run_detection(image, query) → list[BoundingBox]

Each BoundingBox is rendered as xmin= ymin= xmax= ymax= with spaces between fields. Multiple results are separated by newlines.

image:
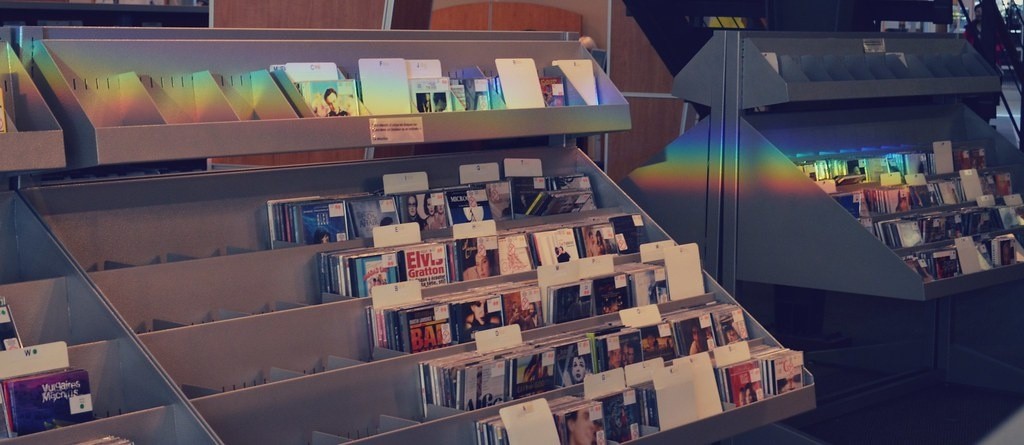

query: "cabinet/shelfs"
xmin=0 ymin=40 xmax=66 ymax=169
xmin=15 ymin=26 xmax=817 ymax=445
xmin=617 ymin=30 xmax=1023 ymax=427
xmin=0 ymin=189 xmax=222 ymax=445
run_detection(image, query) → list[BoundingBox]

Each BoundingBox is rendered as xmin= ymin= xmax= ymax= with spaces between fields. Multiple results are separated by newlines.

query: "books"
xmin=796 ymin=137 xmax=1024 ymax=283
xmin=885 ymin=52 xmax=908 ymax=68
xmin=0 ymin=297 xmax=135 ymax=445
xmin=761 ymin=53 xmax=778 ymax=73
xmin=862 ymin=38 xmax=886 ymax=54
xmin=267 ymin=60 xmax=803 ymax=445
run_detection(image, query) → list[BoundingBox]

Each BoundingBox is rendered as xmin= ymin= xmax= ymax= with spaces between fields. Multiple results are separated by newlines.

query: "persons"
xmin=323 ymin=87 xmax=352 ymax=117
xmin=965 ymin=3 xmax=1024 ymax=73
